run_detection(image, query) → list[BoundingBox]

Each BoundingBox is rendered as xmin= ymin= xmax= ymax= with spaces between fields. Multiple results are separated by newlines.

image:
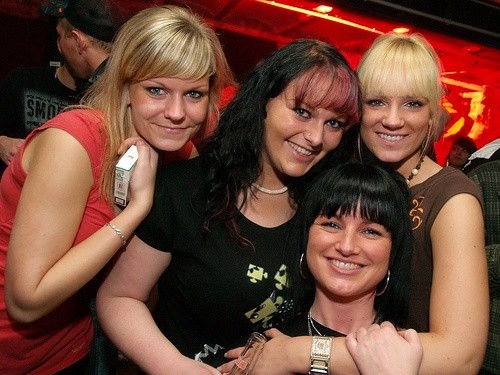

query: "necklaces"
xmin=308 ymin=311 xmax=312 ymax=336
xmin=310 ymin=309 xmax=321 ymax=336
xmin=405 ymin=152 xmax=425 ymax=185
xmin=251 ymin=183 xmax=288 ymax=195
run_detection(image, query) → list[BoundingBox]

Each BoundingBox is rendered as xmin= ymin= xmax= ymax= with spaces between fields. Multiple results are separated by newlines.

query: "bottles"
xmin=228 ymin=332 xmax=267 ymax=375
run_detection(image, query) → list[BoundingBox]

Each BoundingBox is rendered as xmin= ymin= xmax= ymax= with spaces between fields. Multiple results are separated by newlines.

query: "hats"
xmin=39 ymin=1 xmax=117 ymax=42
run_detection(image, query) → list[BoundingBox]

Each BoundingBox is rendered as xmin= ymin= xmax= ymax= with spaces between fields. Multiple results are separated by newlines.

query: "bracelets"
xmin=106 ymin=222 xmax=126 ymax=246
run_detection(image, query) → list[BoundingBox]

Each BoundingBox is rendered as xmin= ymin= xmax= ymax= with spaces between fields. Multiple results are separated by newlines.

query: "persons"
xmin=96 ymin=40 xmax=361 ymax=375
xmin=217 ymin=33 xmax=485 ymax=375
xmin=0 ymin=1 xmax=116 ymax=178
xmin=0 ymin=4 xmax=237 ymax=375
xmin=423 ymin=110 xmax=500 ymax=375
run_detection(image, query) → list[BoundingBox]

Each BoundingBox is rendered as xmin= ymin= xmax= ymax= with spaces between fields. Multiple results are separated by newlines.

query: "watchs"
xmin=309 ymin=336 xmax=334 ymax=375
